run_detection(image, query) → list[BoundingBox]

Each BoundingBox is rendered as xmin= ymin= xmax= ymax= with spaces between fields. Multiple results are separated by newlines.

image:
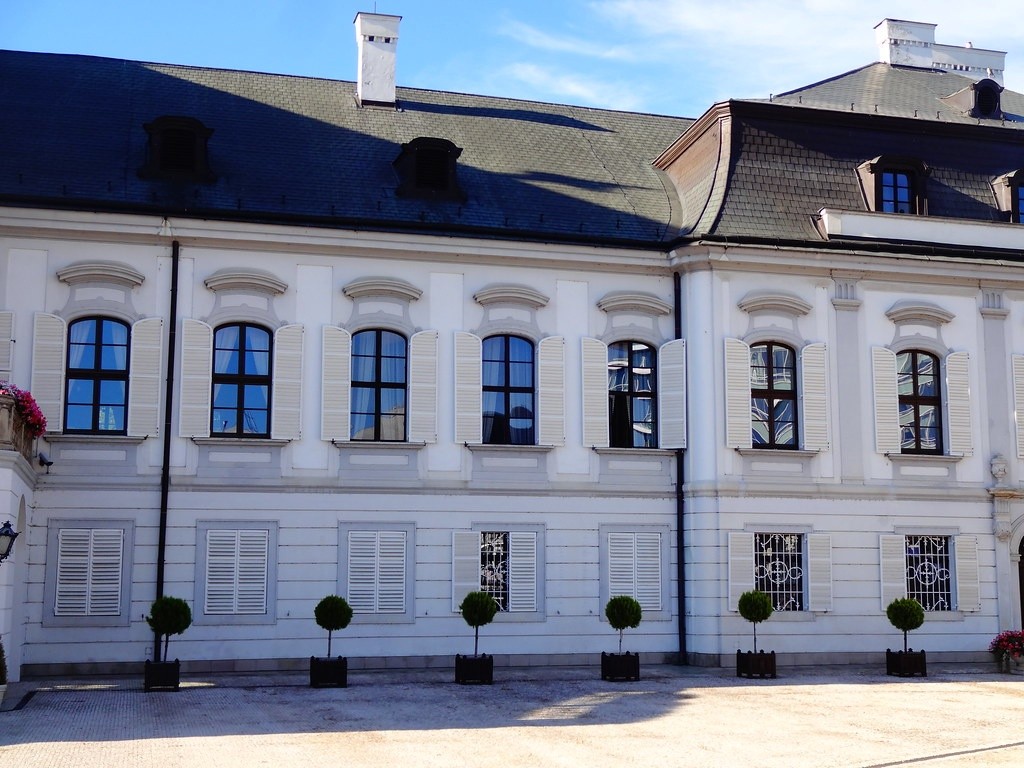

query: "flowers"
xmin=0 ymin=378 xmax=47 ymax=438
xmin=987 ymin=630 xmax=1024 ymax=664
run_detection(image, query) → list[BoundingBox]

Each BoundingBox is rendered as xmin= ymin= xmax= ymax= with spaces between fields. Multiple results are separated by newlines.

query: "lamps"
xmin=0 ymin=519 xmax=22 ymax=567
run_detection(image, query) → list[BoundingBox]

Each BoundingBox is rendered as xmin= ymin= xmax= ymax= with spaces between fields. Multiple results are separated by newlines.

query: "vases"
xmin=0 ymin=394 xmax=33 ymax=465
xmin=1002 ymin=653 xmax=1024 ymax=675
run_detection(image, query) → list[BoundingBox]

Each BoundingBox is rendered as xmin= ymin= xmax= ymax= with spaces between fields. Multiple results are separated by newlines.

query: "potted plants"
xmin=454 ymin=591 xmax=497 ymax=685
xmin=0 ymin=634 xmax=8 ymax=705
xmin=886 ymin=597 xmax=927 ymax=677
xmin=144 ymin=596 xmax=193 ymax=691
xmin=601 ymin=595 xmax=642 ymax=681
xmin=310 ymin=593 xmax=354 ymax=687
xmin=736 ymin=589 xmax=776 ymax=678
